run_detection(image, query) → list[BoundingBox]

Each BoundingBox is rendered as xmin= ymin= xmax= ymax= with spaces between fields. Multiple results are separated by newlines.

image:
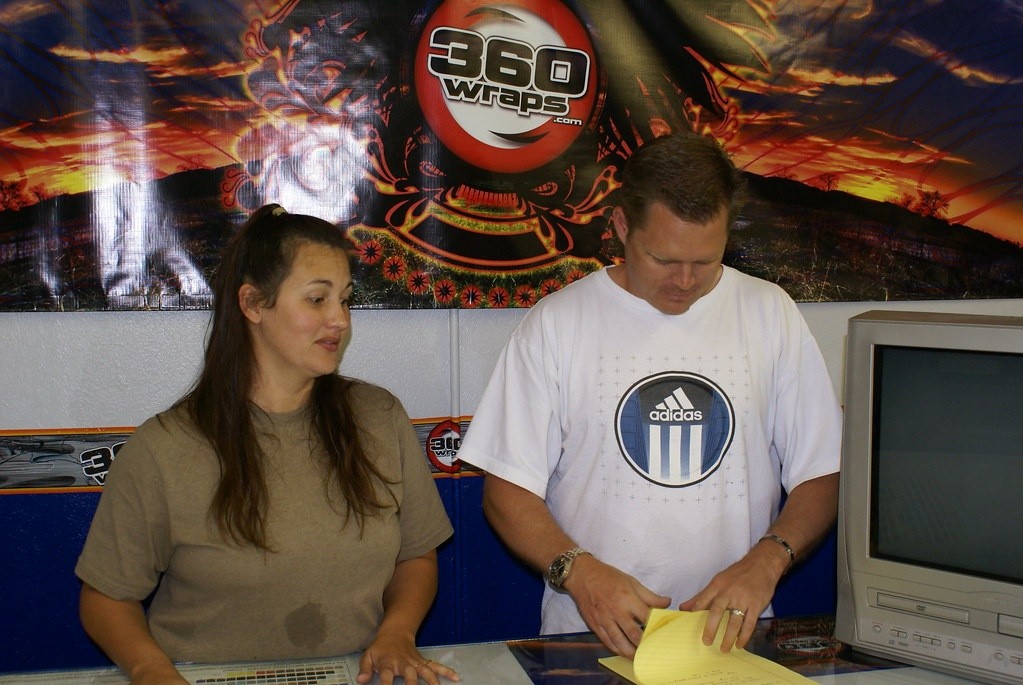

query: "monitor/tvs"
xmin=834 ymin=308 xmax=1023 ymax=685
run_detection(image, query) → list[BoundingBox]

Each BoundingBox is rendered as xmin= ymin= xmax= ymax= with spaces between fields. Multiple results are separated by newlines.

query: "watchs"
xmin=548 ymin=547 xmax=592 ymax=594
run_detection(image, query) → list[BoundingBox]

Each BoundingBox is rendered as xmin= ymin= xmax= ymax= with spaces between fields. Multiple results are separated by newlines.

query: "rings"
xmin=414 ymin=661 xmax=423 ymax=668
xmin=731 ymin=609 xmax=745 ymax=617
xmin=423 ymin=659 xmax=432 ymax=665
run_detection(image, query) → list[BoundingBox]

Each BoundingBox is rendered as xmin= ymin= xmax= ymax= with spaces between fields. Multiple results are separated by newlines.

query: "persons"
xmin=74 ymin=202 xmax=457 ymax=685
xmin=450 ymin=130 xmax=842 ymax=661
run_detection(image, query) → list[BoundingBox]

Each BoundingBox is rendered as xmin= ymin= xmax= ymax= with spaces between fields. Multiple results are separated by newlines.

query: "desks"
xmin=0 ymin=615 xmax=987 ymax=685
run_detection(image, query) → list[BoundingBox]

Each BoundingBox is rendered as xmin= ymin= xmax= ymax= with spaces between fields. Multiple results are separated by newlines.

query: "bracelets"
xmin=759 ymin=534 xmax=794 ymax=566
